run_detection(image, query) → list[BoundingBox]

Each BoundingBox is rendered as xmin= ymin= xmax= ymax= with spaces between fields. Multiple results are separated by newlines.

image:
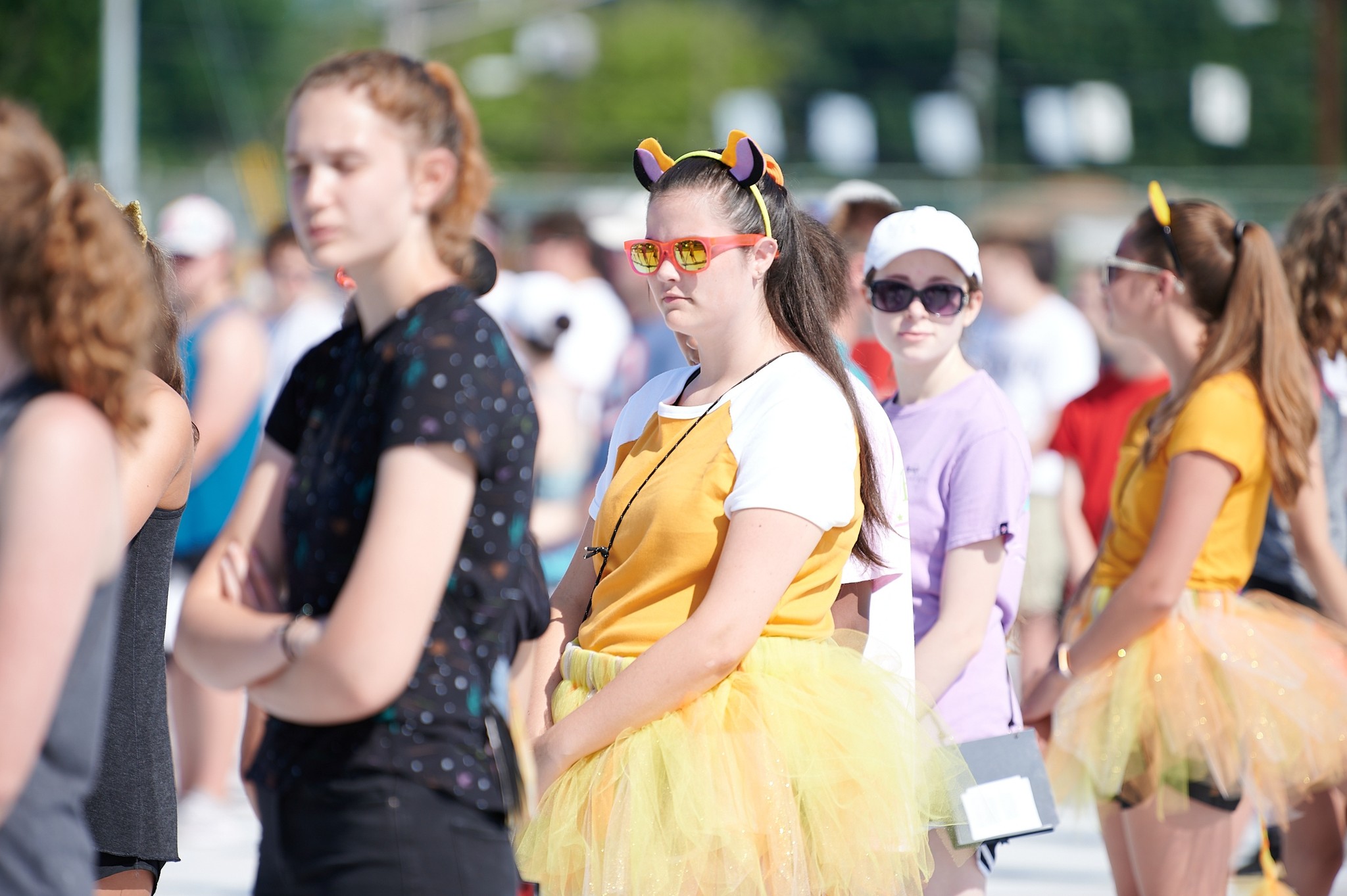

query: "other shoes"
xmin=161 ymin=793 xmax=261 ymax=890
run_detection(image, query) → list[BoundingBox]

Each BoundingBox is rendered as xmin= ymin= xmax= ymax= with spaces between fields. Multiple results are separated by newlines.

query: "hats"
xmin=152 ymin=196 xmax=233 ymax=257
xmin=864 ymin=205 xmax=983 ymax=285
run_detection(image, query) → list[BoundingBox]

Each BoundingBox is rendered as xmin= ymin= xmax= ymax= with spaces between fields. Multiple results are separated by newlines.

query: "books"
xmin=937 ymin=727 xmax=1061 ymax=848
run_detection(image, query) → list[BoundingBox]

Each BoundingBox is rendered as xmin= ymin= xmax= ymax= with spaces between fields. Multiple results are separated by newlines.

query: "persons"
xmin=1228 ymin=177 xmax=1347 ymax=896
xmin=856 ymin=208 xmax=1033 ymax=896
xmin=518 ymin=146 xmax=886 ymax=896
xmin=173 ymin=46 xmax=545 ymax=896
xmin=1012 ymin=197 xmax=1323 ymax=896
xmin=796 ymin=181 xmax=1173 ymax=710
xmin=439 ymin=183 xmax=699 ymax=722
xmin=0 ymin=99 xmax=352 ymax=896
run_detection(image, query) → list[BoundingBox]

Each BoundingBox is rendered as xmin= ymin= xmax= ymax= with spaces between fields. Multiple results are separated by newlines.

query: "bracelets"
xmin=278 ymin=614 xmax=313 ymax=669
xmin=1054 ymin=640 xmax=1079 ymax=683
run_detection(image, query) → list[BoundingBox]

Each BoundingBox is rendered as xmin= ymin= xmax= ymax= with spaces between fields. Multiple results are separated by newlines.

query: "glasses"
xmin=1102 ymin=255 xmax=1190 ymax=300
xmin=870 ymin=279 xmax=972 ymax=318
xmin=625 ymin=231 xmax=778 ymax=279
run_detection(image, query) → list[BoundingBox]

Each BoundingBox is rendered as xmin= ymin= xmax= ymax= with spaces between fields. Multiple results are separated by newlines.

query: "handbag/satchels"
xmin=938 ymin=729 xmax=1061 ymax=850
